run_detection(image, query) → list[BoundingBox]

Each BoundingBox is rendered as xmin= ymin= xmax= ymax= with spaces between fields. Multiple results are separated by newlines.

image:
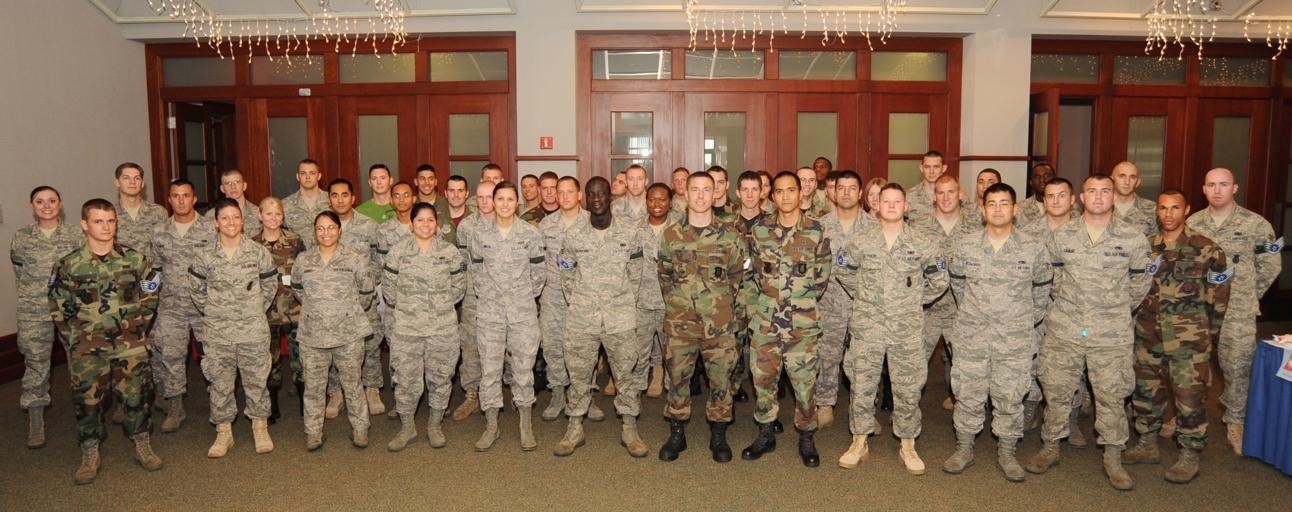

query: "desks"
xmin=1241 ymin=333 xmax=1292 ymax=478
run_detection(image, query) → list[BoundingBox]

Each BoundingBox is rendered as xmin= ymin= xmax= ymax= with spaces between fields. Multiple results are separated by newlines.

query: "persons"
xmin=517 ymin=176 xmax=538 ymax=213
xmin=826 ymin=172 xmax=838 ymax=208
xmin=141 ymin=180 xmax=216 ymax=433
xmin=456 ymin=184 xmax=496 ymax=419
xmin=251 ymin=196 xmax=306 ymax=423
xmin=1123 ymin=190 xmax=1230 ymax=482
xmin=375 ymin=183 xmax=416 ymax=276
xmin=325 ymin=179 xmax=387 ymax=420
xmin=612 ymin=166 xmax=647 ymax=237
xmin=1186 ymin=168 xmax=1282 ymax=454
xmin=705 ymin=166 xmax=736 ymax=217
xmin=819 ymin=169 xmax=877 ymax=426
xmin=756 ymin=171 xmax=776 ymax=214
xmin=522 ymin=171 xmax=556 ymax=227
xmin=1111 ymin=162 xmax=1159 ymax=237
xmin=554 ymin=177 xmax=648 ymax=458
xmin=189 ymin=198 xmax=279 ymax=458
xmin=609 ymin=170 xmax=628 ymax=197
xmin=414 ymin=164 xmax=454 ymax=240
xmin=863 ymin=176 xmax=884 ymax=216
xmin=11 ymin=189 xmax=86 ymax=449
xmin=905 ymin=151 xmax=969 ymax=228
xmin=1027 ymin=174 xmax=1153 ymax=490
xmin=668 ymin=166 xmax=688 ymax=214
xmin=381 ymin=203 xmax=466 ymax=452
xmin=469 ymin=181 xmax=546 ymax=452
xmin=729 ymin=169 xmax=772 ymax=234
xmin=944 ymin=182 xmax=1052 ymax=481
xmin=48 ymin=198 xmax=163 ymax=484
xmin=283 ymin=160 xmax=330 ymax=250
xmin=468 ymin=164 xmax=501 ymax=212
xmin=204 ymin=169 xmax=259 ymax=240
xmin=834 ymin=182 xmax=951 ymax=474
xmin=628 ymin=183 xmax=683 ymax=397
xmin=290 ymin=208 xmax=375 ymax=450
xmin=658 ymin=172 xmax=743 ymax=461
xmin=1021 ymin=177 xmax=1080 ymax=246
xmin=443 ymin=174 xmax=472 ymax=226
xmin=795 ymin=167 xmax=829 ymax=220
xmin=960 ymin=169 xmax=1027 ymax=229
xmin=111 ymin=163 xmax=168 ymax=254
xmin=356 ymin=163 xmax=397 ymax=222
xmin=745 ymin=170 xmax=832 ymax=467
xmin=812 ymin=157 xmax=832 ymax=190
xmin=540 ymin=175 xmax=590 ymax=421
xmin=906 ymin=176 xmax=983 ymax=365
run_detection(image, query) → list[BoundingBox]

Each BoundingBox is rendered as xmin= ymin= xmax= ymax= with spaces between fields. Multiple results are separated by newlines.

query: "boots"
xmin=325 ymin=390 xmax=345 ymax=419
xmin=388 ymin=405 xmax=398 ymax=419
xmin=1164 ymin=447 xmax=1201 ymax=484
xmin=474 ymin=407 xmax=500 ymax=452
xmin=996 ymin=444 xmax=1025 ymax=481
xmin=1120 ymin=432 xmax=1161 ymax=464
xmin=518 ymin=406 xmax=537 ymax=451
xmin=659 ymin=420 xmax=687 ymax=460
xmin=306 ymin=431 xmax=325 ymax=450
xmin=207 ymin=421 xmax=234 ymax=458
xmin=881 ymin=378 xmax=894 ymax=412
xmin=1226 ymin=423 xmax=1246 ymax=455
xmin=817 ymin=405 xmax=834 ymax=429
xmin=269 ymin=389 xmax=280 ymax=422
xmin=542 ymin=387 xmax=567 ymax=419
xmin=943 ymin=432 xmax=976 ymax=473
xmin=349 ymin=429 xmax=368 ymax=447
xmin=689 ymin=380 xmax=701 ymax=396
xmin=160 ymin=396 xmax=187 ymax=432
xmin=27 ymin=407 xmax=46 ymax=448
xmin=452 ymin=388 xmax=480 ymax=421
xmin=1023 ymin=401 xmax=1037 ymax=431
xmin=1159 ymin=415 xmax=1178 ymax=438
xmin=1026 ymin=438 xmax=1060 ymax=473
xmin=75 ymin=439 xmax=102 ymax=484
xmin=752 ymin=417 xmax=783 ymax=433
xmin=365 ymin=387 xmax=385 ymax=415
xmin=742 ymin=422 xmax=776 ymax=460
xmin=586 ymin=399 xmax=604 ymax=421
xmin=426 ymin=407 xmax=446 ymax=448
xmin=1068 ymin=407 xmax=1087 ymax=448
xmin=621 ymin=414 xmax=648 ymax=457
xmin=646 ymin=366 xmax=664 ymax=397
xmin=898 ymin=438 xmax=925 ymax=475
xmin=838 ymin=434 xmax=869 ymax=468
xmin=387 ymin=412 xmax=418 ymax=451
xmin=734 ymin=388 xmax=747 ymax=401
xmin=1103 ymin=444 xmax=1133 ymax=489
xmin=111 ymin=401 xmax=123 ymax=421
xmin=604 ymin=376 xmax=616 ymax=395
xmin=799 ymin=430 xmax=819 ymax=466
xmin=251 ymin=418 xmax=274 ymax=453
xmin=129 ymin=432 xmax=163 ymax=470
xmin=153 ymin=392 xmax=185 ymax=419
xmin=553 ymin=415 xmax=586 ymax=456
xmin=709 ymin=422 xmax=731 ymax=462
